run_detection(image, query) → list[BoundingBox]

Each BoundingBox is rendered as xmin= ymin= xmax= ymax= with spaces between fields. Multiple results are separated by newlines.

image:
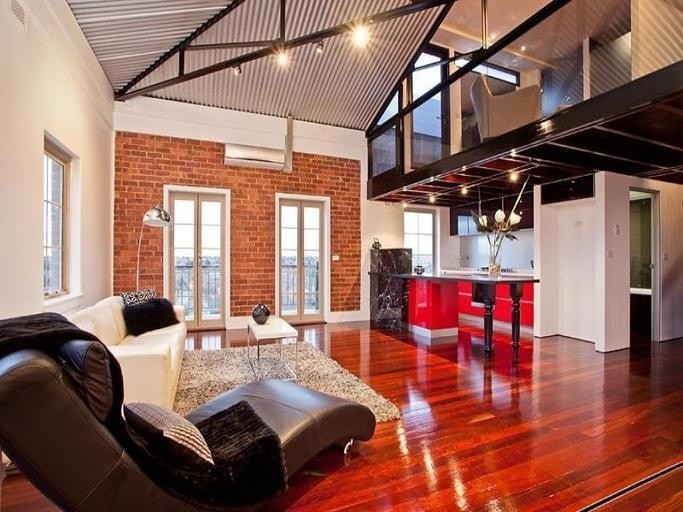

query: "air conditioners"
xmin=223 ymin=143 xmax=286 ymax=172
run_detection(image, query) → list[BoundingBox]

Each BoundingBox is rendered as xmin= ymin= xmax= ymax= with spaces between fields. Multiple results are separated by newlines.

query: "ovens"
xmin=471 ymin=281 xmax=496 ymax=309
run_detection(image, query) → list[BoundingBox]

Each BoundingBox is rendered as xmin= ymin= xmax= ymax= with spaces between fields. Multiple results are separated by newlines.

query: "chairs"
xmin=0 ymin=312 xmax=376 ymax=511
xmin=470 ymin=73 xmax=541 ymax=144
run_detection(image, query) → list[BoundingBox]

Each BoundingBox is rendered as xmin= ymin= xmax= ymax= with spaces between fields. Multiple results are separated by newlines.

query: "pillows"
xmin=124 ymin=402 xmax=216 ymax=477
xmin=121 ymin=287 xmax=159 ymax=308
xmin=122 ymin=298 xmax=180 ymax=337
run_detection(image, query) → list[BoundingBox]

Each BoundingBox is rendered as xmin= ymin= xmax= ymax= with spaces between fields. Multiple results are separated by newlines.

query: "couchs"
xmin=67 ymin=295 xmax=187 ymax=422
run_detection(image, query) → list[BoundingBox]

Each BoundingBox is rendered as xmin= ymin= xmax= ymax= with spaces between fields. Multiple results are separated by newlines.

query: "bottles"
xmin=414 ymin=266 xmax=425 ymax=275
xmin=372 ymin=242 xmax=381 ymax=250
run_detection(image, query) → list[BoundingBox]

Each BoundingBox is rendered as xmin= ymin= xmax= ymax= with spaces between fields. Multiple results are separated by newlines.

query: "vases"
xmin=487 ymin=237 xmax=502 ymax=278
xmin=251 ymin=303 xmax=270 ymax=324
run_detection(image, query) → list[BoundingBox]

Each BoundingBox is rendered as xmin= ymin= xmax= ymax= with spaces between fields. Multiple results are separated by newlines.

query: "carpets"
xmin=173 ymin=342 xmax=402 ymax=425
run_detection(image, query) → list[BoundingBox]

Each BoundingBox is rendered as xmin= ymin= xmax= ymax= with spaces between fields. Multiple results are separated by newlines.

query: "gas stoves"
xmin=474 ymin=267 xmax=513 ymax=272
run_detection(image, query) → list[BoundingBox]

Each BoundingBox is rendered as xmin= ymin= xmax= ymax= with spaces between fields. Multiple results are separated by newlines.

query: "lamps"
xmin=135 ymin=199 xmax=172 ymax=293
xmin=317 ymin=39 xmax=324 ymax=53
xmin=235 ymin=64 xmax=241 ymax=75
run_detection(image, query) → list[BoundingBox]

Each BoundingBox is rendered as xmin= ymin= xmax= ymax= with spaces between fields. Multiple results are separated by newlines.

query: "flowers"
xmin=470 ymin=176 xmax=530 ymax=267
